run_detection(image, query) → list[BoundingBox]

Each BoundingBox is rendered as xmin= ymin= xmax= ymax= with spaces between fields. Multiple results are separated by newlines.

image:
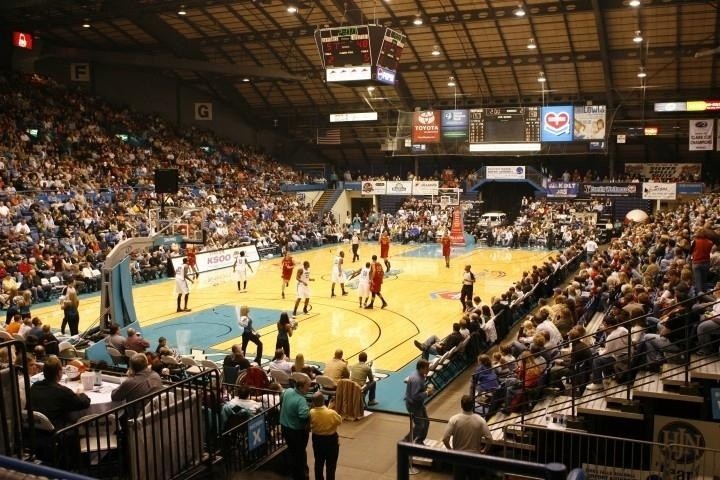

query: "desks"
xmin=30 ymin=370 xmax=126 ymax=407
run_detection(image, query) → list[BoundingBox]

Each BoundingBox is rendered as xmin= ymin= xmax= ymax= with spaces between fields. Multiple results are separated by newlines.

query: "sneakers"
xmin=696 ymin=350 xmax=713 ymax=356
xmin=360 ymin=305 xmax=362 ymax=308
xmin=388 ymin=262 xmax=390 ymax=267
xmin=177 ymin=309 xmax=184 ymax=312
xmin=642 ymin=363 xmax=656 ymax=371
xmin=342 ymin=292 xmax=348 ymax=295
xmin=184 ymin=308 xmax=191 ymax=311
xmin=387 ymin=266 xmax=389 ymax=272
xmin=543 ymin=387 xmax=560 ymax=393
xmin=352 ymin=260 xmax=355 ymax=262
xmin=303 ymin=309 xmax=309 ymax=314
xmin=381 ymin=303 xmax=387 ymax=309
xmin=357 ymin=255 xmax=359 ymax=259
xmin=286 ymin=283 xmax=288 ymax=287
xmin=447 ymin=263 xmax=449 ymax=268
xmin=243 ymin=289 xmax=247 ymax=292
xmin=331 ymin=294 xmax=336 ymax=298
xmin=238 ymin=290 xmax=243 ymax=293
xmin=255 ymin=358 xmax=262 ymax=366
xmin=446 ymin=263 xmax=447 ymax=268
xmin=365 ymin=304 xmax=373 ymax=309
xmin=282 ymin=293 xmax=285 ymax=299
xmin=292 ymin=313 xmax=296 ymax=318
xmin=364 ymin=302 xmax=367 ymax=306
xmin=368 ymin=400 xmax=378 ymax=406
xmin=586 ymin=382 xmax=605 ymax=390
xmin=463 ymin=304 xmax=467 ymax=312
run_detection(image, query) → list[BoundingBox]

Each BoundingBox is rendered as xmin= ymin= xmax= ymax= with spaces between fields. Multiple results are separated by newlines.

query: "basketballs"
xmin=437 ymin=239 xmax=440 ymax=242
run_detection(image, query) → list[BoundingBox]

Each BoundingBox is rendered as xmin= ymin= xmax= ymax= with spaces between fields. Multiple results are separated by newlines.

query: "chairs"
xmin=12 ymin=333 xmax=24 ymax=340
xmin=38 ymin=196 xmax=48 ymax=200
xmin=403 ymin=376 xmax=409 ymax=400
xmin=196 ymin=359 xmax=224 ymax=378
xmin=181 ymin=358 xmax=208 ymax=377
xmin=92 ymin=268 xmax=101 ymax=278
xmin=41 ymin=277 xmax=56 ymax=295
xmin=424 ymin=357 xmax=441 ymax=393
xmin=432 ymin=346 xmax=460 ymax=378
xmin=107 ymin=348 xmax=129 ymax=368
xmin=57 ymin=195 xmax=66 ymax=201
xmin=315 ymin=375 xmax=336 ymax=400
xmin=436 ymin=336 xmax=470 ymax=370
xmin=0 ymin=330 xmax=11 ymax=341
xmin=471 ymin=247 xmax=587 ymax=357
xmin=161 ymin=355 xmax=188 ymax=379
xmin=50 ymin=276 xmax=67 ymax=294
xmin=271 ymin=370 xmax=291 ymax=388
xmin=336 ymin=379 xmax=368 ymax=409
xmin=291 ymin=372 xmax=315 ymax=393
xmin=82 ymin=268 xmax=92 ymax=280
xmin=429 ymin=352 xmax=454 ymax=386
xmin=21 ymin=409 xmax=56 ymax=453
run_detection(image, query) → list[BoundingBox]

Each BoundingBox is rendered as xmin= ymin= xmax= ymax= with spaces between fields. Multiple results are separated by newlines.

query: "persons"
xmin=294 ymin=260 xmax=315 ymax=314
xmin=0 ymin=189 xmax=83 ymax=352
xmin=520 ymin=194 xmax=612 ymax=217
xmin=280 ymin=374 xmax=310 ymax=477
xmin=154 ymin=112 xmax=176 ymax=171
xmin=350 ymin=353 xmax=378 ymax=406
xmin=282 ymin=251 xmax=295 ymax=298
xmin=323 ymin=349 xmax=349 ymax=404
xmin=177 ymin=121 xmax=328 ymax=183
xmin=351 ymin=263 xmax=372 ymax=308
xmin=165 ymin=185 xmax=200 ymax=245
xmin=239 ymin=307 xmax=263 ymax=366
xmin=123 ymin=329 xmax=154 ymax=360
xmin=401 ymin=359 xmax=432 ymax=447
xmin=233 ymin=251 xmax=254 ymax=292
xmin=470 ymin=290 xmax=716 ymax=413
xmin=276 ymin=313 xmax=293 ymax=359
xmin=378 ymin=232 xmax=390 ymax=274
xmin=24 ymin=357 xmax=91 ymax=468
xmin=688 ymin=229 xmax=711 ymax=292
xmin=332 ymin=251 xmax=349 ymax=296
xmin=175 ymin=258 xmax=194 ymax=312
xmin=158 ymin=338 xmax=166 ymax=352
xmin=351 ymin=232 xmax=360 ymax=262
xmin=162 ymin=347 xmax=180 ymax=368
xmin=221 ymin=385 xmax=280 ymax=428
xmin=291 ymin=355 xmax=322 ymax=378
xmin=511 ymin=218 xmax=687 ymax=289
xmin=341 ymin=200 xmax=453 ymax=241
xmin=197 ymin=183 xmax=343 ymax=253
xmin=308 ymin=392 xmax=342 ymax=479
xmin=269 ymin=350 xmax=294 ymax=381
xmin=441 ymin=232 xmax=454 ymax=267
xmin=111 ymin=353 xmax=163 ymax=422
xmin=472 ymin=220 xmax=514 ymax=246
xmin=105 ymin=325 xmax=125 ymax=361
xmin=542 ymin=168 xmax=714 ymax=184
xmin=413 ymin=311 xmax=479 ymax=364
xmin=331 ymin=165 xmax=481 ymax=192
xmin=197 ymin=379 xmax=228 ymax=412
xmin=688 ymin=191 xmax=720 ymax=229
xmin=573 ymin=118 xmax=604 ymax=138
xmin=223 ymin=346 xmax=259 ymax=374
xmin=442 ymin=394 xmax=492 ymax=479
xmin=460 ymin=265 xmax=475 ymax=311
xmin=710 ymin=229 xmax=720 ymax=298
xmin=651 ymin=200 xmax=688 ymax=220
xmin=184 ymin=246 xmax=199 ymax=281
xmin=366 ymin=255 xmax=387 ymax=309
xmin=83 ymin=188 xmax=169 ymax=288
xmin=1 ymin=61 xmax=154 ymax=188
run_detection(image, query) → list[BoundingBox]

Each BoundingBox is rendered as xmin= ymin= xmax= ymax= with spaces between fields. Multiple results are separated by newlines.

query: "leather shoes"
xmin=414 ymin=340 xmax=424 ymax=351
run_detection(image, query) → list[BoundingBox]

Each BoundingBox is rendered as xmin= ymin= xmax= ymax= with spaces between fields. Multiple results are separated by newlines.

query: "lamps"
xmin=447 ymin=76 xmax=456 ymax=87
xmin=177 ymin=4 xmax=187 ymax=15
xmin=367 ymin=86 xmax=375 ymax=91
xmin=636 ymin=66 xmax=647 ymax=78
xmin=527 ymin=37 xmax=536 ymax=49
xmin=632 ymin=30 xmax=643 ymax=43
xmin=432 ymin=45 xmax=441 ymax=56
xmin=81 ymin=23 xmax=92 ymax=28
xmin=286 ymin=2 xmax=297 ymax=13
xmin=414 ymin=19 xmax=422 ymax=25
xmin=537 ymin=71 xmax=546 ymax=82
xmin=384 ymin=0 xmax=392 ymax=2
xmin=515 ymin=4 xmax=525 ymax=17
xmin=629 ymin=0 xmax=641 ymax=7
xmin=242 ymin=77 xmax=250 ymax=82
